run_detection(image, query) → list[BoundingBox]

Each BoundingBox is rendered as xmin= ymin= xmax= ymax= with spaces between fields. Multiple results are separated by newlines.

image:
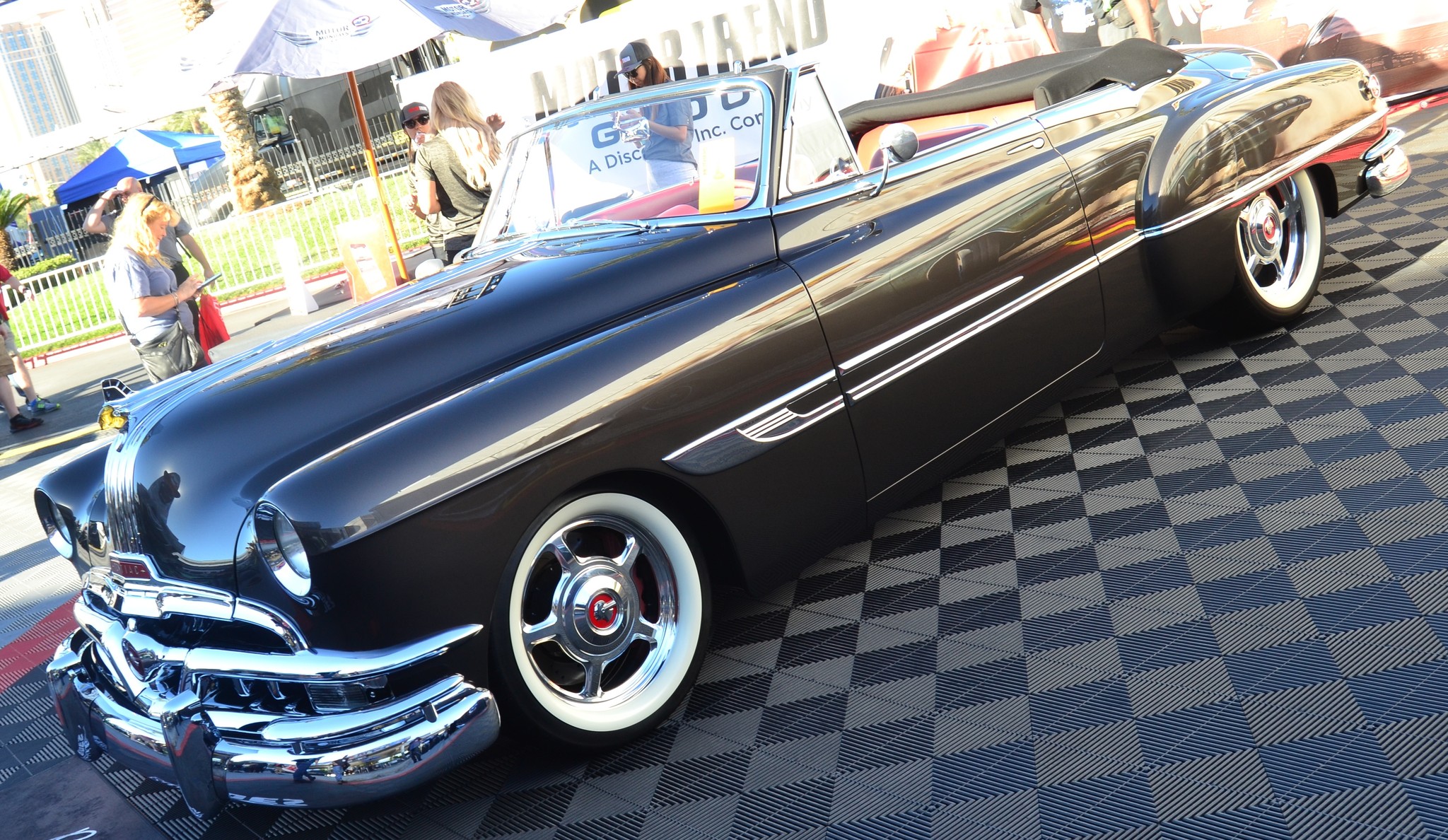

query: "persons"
xmin=81 ymin=177 xmax=216 ymax=368
xmin=147 ymin=470 xmax=182 ymax=523
xmin=398 ymin=101 xmax=506 ymax=267
xmin=102 ymin=193 xmax=213 ymax=387
xmin=0 ymin=256 xmax=60 ymax=415
xmin=412 ymin=82 xmax=504 ymax=268
xmin=609 ymin=41 xmax=700 ymax=189
xmin=0 ymin=319 xmax=44 ymax=433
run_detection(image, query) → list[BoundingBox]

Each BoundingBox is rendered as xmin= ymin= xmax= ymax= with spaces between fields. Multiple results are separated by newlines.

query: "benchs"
xmin=854 ymin=102 xmax=1036 ymax=180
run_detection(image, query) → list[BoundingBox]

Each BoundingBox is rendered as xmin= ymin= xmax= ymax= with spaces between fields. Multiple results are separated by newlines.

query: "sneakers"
xmin=26 ymin=397 xmax=50 ymax=411
xmin=30 ymin=400 xmax=61 ymax=416
xmin=9 ymin=415 xmax=44 ymax=433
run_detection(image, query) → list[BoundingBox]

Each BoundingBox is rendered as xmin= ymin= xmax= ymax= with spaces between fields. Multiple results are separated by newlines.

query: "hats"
xmin=399 ymin=102 xmax=430 ymax=129
xmin=613 ymin=43 xmax=653 ymax=78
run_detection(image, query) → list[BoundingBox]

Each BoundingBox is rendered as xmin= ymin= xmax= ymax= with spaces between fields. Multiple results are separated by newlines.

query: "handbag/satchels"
xmin=196 ymin=287 xmax=231 ymax=350
xmin=134 ymin=319 xmax=204 ymax=385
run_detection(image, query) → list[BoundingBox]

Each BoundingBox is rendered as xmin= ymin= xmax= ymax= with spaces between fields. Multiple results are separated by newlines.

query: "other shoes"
xmin=0 ymin=401 xmax=20 ymax=410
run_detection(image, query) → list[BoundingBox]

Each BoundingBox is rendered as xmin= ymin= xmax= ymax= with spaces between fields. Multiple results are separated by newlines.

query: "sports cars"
xmin=23 ymin=36 xmax=1405 ymax=812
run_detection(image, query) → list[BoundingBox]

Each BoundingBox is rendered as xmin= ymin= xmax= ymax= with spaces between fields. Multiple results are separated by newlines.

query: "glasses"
xmin=622 ymin=63 xmax=643 ymax=80
xmin=403 ymin=115 xmax=430 ymax=129
xmin=141 ymin=194 xmax=162 ymax=216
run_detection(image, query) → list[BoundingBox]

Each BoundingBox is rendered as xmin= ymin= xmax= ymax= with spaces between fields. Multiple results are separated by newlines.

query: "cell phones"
xmin=196 ymin=272 xmax=222 ymax=292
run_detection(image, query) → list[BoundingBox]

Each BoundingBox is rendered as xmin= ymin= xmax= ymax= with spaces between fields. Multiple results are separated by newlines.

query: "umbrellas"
xmin=52 ymin=128 xmax=227 ymax=207
xmin=201 ymin=0 xmax=594 ymax=282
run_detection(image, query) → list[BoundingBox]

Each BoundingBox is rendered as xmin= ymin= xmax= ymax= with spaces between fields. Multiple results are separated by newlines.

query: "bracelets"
xmin=172 ymin=290 xmax=180 ymax=307
